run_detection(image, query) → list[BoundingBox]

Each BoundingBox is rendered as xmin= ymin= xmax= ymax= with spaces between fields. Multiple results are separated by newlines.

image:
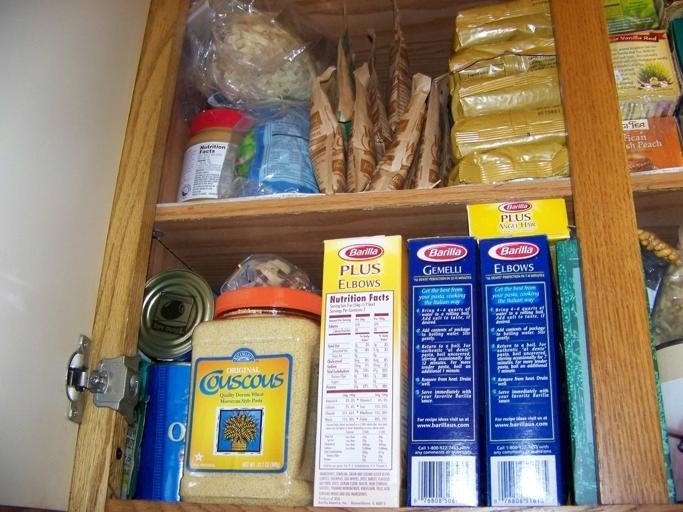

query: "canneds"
xmin=136 ymin=269 xmax=217 ymax=363
xmin=128 ymin=362 xmax=192 ymax=502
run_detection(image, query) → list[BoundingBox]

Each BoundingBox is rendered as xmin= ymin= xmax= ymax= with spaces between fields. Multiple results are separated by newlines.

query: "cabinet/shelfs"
xmin=59 ymin=0 xmax=682 ymax=511
